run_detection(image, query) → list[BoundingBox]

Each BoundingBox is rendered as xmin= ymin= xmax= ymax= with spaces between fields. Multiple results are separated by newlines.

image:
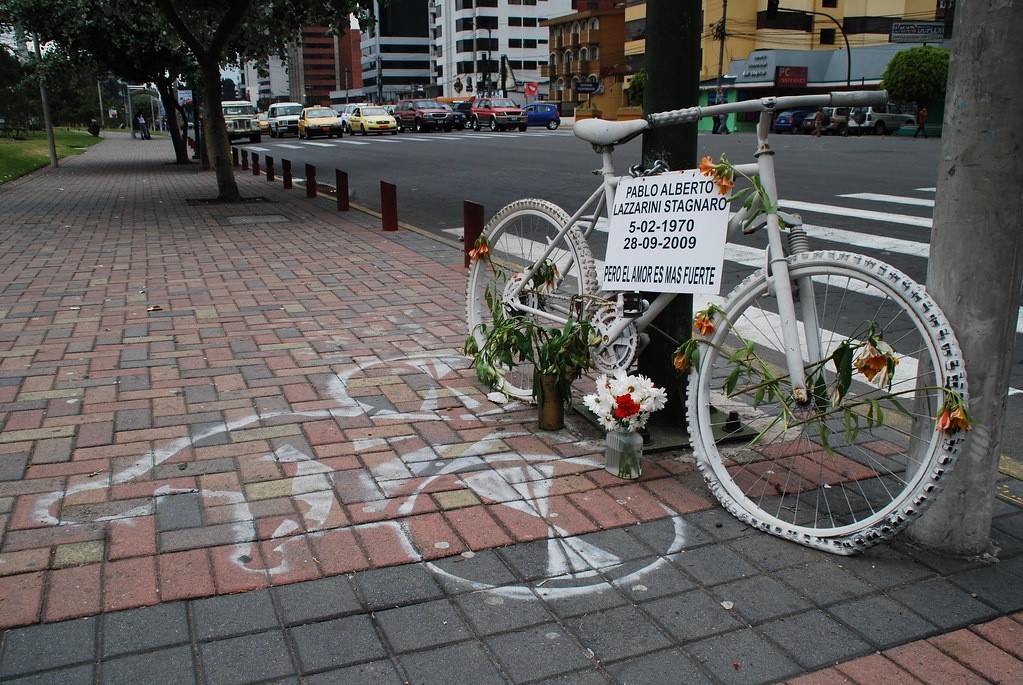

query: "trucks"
xmin=221 ymin=101 xmax=261 ymax=144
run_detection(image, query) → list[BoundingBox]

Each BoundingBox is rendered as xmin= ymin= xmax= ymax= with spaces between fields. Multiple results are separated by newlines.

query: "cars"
xmin=449 ymin=102 xmax=472 ymax=129
xmin=297 ymin=105 xmax=343 ymax=140
xmin=818 ymin=107 xmax=851 ymax=136
xmin=188 ymin=122 xmax=194 ymax=129
xmin=153 ymin=119 xmax=169 ymax=132
xmin=847 ymin=102 xmax=917 ymax=135
xmin=802 ymin=109 xmax=819 ymax=133
xmin=257 ymin=114 xmax=269 ymax=132
xmin=382 ymin=105 xmax=396 ymax=113
xmin=440 ymin=103 xmax=465 ymax=132
xmin=348 ymin=106 xmax=398 ymax=136
xmin=521 ymin=103 xmax=560 ymax=130
xmin=775 ymin=109 xmax=814 ymax=134
xmin=339 ymin=103 xmax=370 ymax=133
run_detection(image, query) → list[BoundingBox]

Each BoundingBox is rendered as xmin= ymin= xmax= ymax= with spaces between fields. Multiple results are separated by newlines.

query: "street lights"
xmin=709 ymin=21 xmax=726 ymax=86
xmin=469 ymin=21 xmax=491 ymax=80
xmin=363 ymin=54 xmax=382 ymax=102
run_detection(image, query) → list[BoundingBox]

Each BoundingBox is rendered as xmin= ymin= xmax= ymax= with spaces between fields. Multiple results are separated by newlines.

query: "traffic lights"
xmin=767 ymin=0 xmax=780 ymax=21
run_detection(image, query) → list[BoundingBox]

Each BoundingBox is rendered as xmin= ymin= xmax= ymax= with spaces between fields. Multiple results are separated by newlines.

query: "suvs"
xmin=469 ymin=97 xmax=527 ymax=132
xmin=391 ymin=99 xmax=452 ymax=133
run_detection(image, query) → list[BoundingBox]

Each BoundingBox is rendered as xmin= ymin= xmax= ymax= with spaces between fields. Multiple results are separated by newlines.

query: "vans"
xmin=268 ymin=102 xmax=304 ymax=138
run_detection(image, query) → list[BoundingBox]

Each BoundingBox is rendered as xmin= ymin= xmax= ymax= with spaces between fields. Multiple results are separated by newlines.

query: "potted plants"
xmin=464 ymin=313 xmax=601 ymax=430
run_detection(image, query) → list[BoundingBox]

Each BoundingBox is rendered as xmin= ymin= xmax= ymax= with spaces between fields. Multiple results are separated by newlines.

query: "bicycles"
xmin=465 ymin=91 xmax=969 ymax=555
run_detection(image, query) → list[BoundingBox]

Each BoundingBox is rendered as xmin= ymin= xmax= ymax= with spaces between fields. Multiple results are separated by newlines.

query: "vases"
xmin=606 ymin=426 xmax=643 ymax=479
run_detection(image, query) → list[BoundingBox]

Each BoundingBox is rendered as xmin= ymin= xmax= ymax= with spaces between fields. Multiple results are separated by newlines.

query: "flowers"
xmin=582 ymin=369 xmax=669 ymax=431
xmin=698 ymin=151 xmax=787 ymax=230
xmin=467 ymin=235 xmax=561 ymax=311
xmin=671 ymin=301 xmax=977 ymax=461
